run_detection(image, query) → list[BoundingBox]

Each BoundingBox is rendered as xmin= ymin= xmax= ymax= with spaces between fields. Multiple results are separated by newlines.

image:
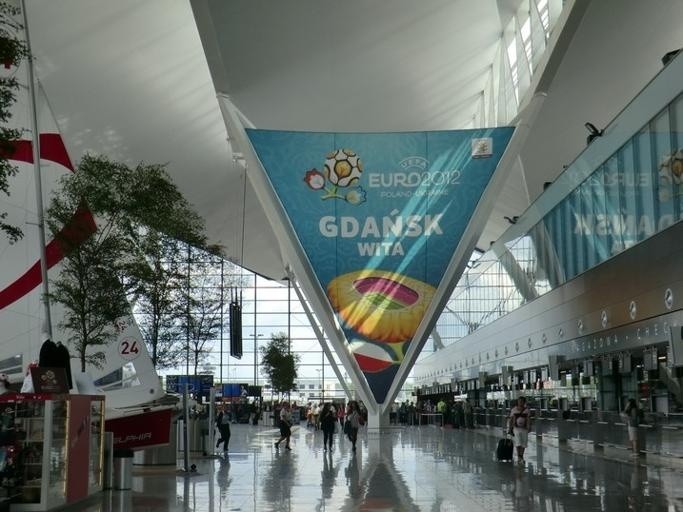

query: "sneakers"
xmin=285 ymin=446 xmax=291 ymax=450
xmin=629 ymin=453 xmax=640 ymax=458
xmin=517 ymin=459 xmax=525 ymax=465
xmin=630 ymin=419 xmax=638 ymax=428
xmin=275 ymin=443 xmax=278 ymax=449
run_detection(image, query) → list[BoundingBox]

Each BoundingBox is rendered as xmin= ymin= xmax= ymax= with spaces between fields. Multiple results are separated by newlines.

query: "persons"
xmin=211 ymin=396 xmax=474 ymax=453
xmin=319 ymin=450 xmax=337 ymax=498
xmin=624 ymin=398 xmax=640 ymax=457
xmin=508 ymin=397 xmax=531 ymax=465
xmin=625 ymin=458 xmax=643 ymax=512
xmin=509 ymin=469 xmax=534 ymax=512
xmin=215 ymin=453 xmax=232 ymax=492
xmin=344 ymin=453 xmax=362 ymax=499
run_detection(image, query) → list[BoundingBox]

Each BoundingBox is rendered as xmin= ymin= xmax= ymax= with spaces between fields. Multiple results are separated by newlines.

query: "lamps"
xmin=503 ymin=216 xmax=519 ymax=225
xmin=584 ymin=121 xmax=603 ymax=140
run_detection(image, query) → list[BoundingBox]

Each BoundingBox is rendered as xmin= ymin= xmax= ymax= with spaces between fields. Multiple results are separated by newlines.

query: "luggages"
xmin=495 ymin=426 xmax=513 ymax=461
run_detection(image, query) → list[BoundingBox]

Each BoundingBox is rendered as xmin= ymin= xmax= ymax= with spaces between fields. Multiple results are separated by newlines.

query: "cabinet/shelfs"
xmin=1 ymin=392 xmax=66 ymax=510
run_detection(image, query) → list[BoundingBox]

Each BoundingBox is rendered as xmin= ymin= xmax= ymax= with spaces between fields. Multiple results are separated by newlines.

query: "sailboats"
xmin=0 ymin=50 xmax=174 ymax=455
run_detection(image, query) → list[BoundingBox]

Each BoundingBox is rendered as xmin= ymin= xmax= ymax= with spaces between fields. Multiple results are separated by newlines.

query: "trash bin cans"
xmin=263 ymin=411 xmax=274 ymax=426
xmin=112 ymin=448 xmax=133 ymax=490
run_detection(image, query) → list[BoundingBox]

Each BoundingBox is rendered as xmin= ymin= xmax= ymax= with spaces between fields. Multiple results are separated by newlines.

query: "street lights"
xmin=315 ymin=368 xmax=323 ymax=399
xmin=249 ymin=332 xmax=263 ymax=398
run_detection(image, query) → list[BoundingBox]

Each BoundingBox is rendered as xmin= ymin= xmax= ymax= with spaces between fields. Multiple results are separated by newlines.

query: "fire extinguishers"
xmin=5 ymin=444 xmax=15 ymax=468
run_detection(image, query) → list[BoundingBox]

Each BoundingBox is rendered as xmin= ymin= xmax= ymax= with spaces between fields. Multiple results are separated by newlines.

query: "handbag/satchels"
xmin=344 ymin=415 xmax=352 ymax=434
xmin=507 ymin=426 xmax=515 ymax=436
